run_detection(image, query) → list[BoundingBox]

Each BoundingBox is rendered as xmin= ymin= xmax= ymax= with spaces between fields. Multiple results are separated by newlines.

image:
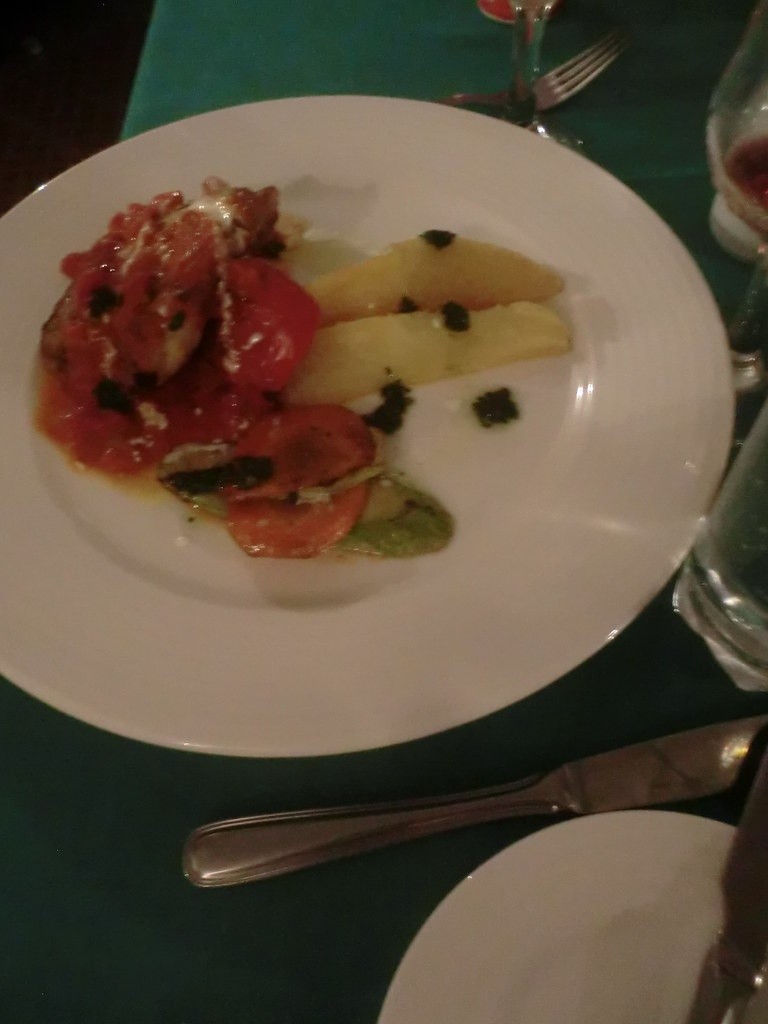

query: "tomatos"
xmin=227 ymin=401 xmax=378 ymax=563
xmin=203 ymin=258 xmax=321 ymax=399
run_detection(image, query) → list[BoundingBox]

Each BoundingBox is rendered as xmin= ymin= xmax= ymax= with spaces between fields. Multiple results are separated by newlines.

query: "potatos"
xmin=273 ymin=231 xmax=579 ymax=410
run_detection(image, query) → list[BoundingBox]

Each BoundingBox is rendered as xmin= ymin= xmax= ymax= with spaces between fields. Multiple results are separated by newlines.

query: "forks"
xmin=431 ymin=37 xmax=628 ymax=114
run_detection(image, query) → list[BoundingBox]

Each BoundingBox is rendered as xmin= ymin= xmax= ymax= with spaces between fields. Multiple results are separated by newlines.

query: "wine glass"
xmin=708 ymin=229 xmax=768 ymax=449
xmin=481 ymin=0 xmax=585 ymax=158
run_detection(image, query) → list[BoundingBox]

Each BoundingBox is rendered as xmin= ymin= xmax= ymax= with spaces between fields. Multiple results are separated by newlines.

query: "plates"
xmin=377 ymin=810 xmax=768 ymax=1024
xmin=0 ymin=91 xmax=733 ymax=761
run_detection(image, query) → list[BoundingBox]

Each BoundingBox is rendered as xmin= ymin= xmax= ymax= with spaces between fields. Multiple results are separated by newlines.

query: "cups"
xmin=699 ymin=3 xmax=768 ymax=267
xmin=671 ymin=390 xmax=768 ymax=701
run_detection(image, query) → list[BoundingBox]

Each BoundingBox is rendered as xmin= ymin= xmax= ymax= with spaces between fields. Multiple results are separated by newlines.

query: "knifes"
xmin=688 ymin=747 xmax=767 ymax=1022
xmin=179 ymin=715 xmax=767 ymax=909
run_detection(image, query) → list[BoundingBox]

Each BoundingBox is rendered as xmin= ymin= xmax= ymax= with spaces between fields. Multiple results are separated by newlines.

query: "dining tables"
xmin=1 ymin=0 xmax=768 ymax=1024
